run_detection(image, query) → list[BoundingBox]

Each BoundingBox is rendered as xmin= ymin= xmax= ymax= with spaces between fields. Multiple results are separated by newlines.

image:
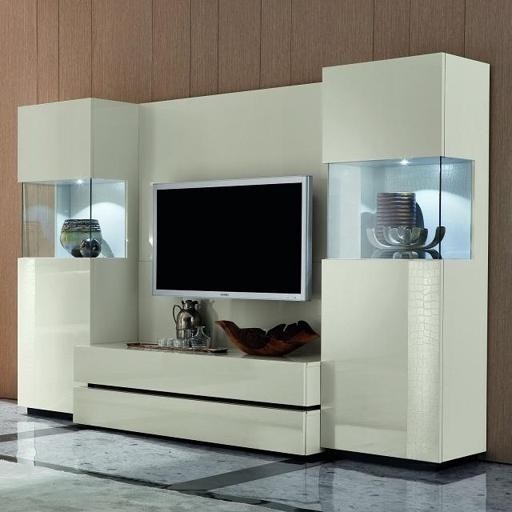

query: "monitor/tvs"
xmin=150 ymin=174 xmax=314 ymax=303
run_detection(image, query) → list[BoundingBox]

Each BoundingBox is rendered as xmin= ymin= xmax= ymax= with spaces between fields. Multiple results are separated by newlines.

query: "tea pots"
xmin=172 ymin=299 xmax=202 ymax=340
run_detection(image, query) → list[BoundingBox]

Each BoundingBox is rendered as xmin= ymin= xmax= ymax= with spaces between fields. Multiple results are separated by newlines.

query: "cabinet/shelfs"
xmin=17 ymin=51 xmax=490 ymax=463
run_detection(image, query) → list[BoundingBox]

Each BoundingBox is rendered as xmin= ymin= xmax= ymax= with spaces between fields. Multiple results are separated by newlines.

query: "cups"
xmin=173 ymin=340 xmax=183 ymax=349
xmin=167 ymin=338 xmax=173 ymax=348
xmin=183 ymin=340 xmax=189 ymax=349
